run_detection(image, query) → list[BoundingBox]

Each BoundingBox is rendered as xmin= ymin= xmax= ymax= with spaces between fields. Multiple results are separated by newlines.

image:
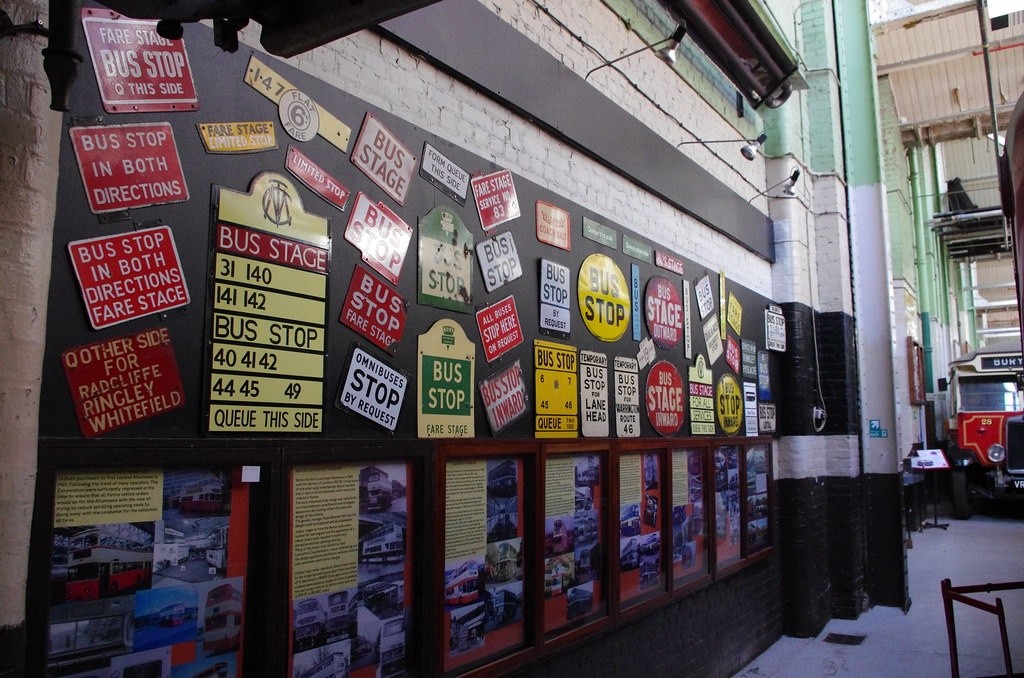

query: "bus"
xmin=64 ymin=445 xmax=768 ymax=676
xmin=937 ymin=342 xmax=1024 ymax=521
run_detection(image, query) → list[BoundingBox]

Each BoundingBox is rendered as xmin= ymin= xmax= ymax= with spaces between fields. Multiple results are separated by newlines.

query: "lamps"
xmin=747 ymin=169 xmax=801 ymax=204
xmin=584 ymin=20 xmax=687 ymax=82
xmin=677 ymin=131 xmax=769 ymax=163
